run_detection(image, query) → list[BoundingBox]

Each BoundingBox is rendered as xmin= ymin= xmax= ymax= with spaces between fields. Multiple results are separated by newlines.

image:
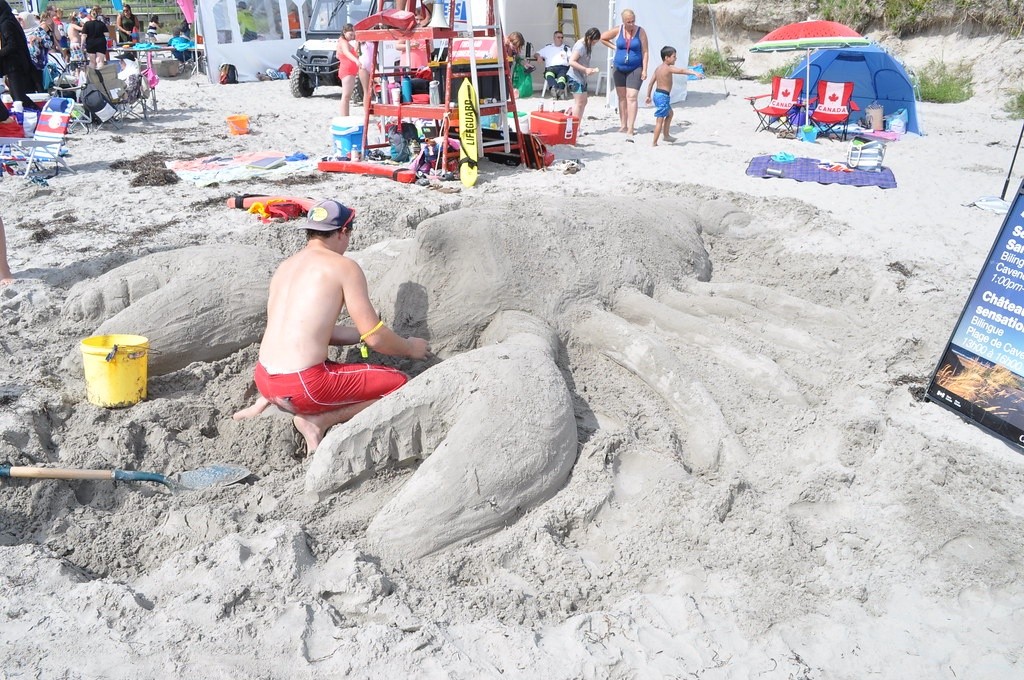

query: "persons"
xmin=237 ymin=1 xmax=258 ymax=42
xmin=0 ymin=217 xmax=16 ymax=285
xmin=600 ymin=8 xmax=649 ymax=136
xmin=533 ymin=31 xmax=572 ymax=100
xmin=396 ymin=8 xmax=435 ymax=70
xmin=354 ymin=40 xmax=380 ymax=113
xmin=0 ymin=0 xmax=141 ymax=139
xmin=168 ymin=17 xmax=200 ymax=63
xmin=646 ymin=46 xmax=703 ymax=146
xmin=504 ymin=32 xmax=534 ymax=71
xmin=231 ymin=201 xmax=434 ymax=458
xmin=147 ymin=15 xmax=161 ymax=43
xmin=334 ymin=23 xmax=363 ymax=116
xmin=564 ymin=27 xmax=601 ymax=132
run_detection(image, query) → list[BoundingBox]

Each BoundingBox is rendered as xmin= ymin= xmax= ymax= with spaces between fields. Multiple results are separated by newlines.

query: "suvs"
xmin=290 ymin=0 xmax=398 ymax=105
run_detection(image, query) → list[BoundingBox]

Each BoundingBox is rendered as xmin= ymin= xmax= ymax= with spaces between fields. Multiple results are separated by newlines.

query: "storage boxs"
xmin=329 ymin=116 xmax=364 ymax=157
xmin=0 ymin=92 xmax=50 ymax=111
xmin=431 ymin=62 xmax=510 ymax=104
xmin=530 ymin=111 xmax=580 ymax=146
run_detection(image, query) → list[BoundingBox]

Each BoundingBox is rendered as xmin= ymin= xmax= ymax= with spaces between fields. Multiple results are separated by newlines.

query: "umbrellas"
xmin=748 ymin=17 xmax=870 ymax=126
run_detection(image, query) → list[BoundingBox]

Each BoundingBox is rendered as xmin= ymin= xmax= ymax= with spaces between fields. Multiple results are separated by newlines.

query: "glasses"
xmin=556 ymin=36 xmax=563 ymax=39
xmin=341 ymin=207 xmax=355 ymax=231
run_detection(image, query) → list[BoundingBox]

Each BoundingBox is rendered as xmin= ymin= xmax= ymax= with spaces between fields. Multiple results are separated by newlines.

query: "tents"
xmin=776 ymin=46 xmax=921 ymax=136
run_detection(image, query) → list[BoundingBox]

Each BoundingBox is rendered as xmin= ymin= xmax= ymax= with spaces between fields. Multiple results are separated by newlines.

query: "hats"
xmin=297 ymin=199 xmax=353 ymax=231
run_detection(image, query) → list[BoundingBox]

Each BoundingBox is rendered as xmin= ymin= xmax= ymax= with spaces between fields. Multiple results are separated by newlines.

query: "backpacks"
xmin=218 ymin=63 xmax=240 ymax=85
xmin=526 ymin=130 xmax=554 ymax=167
xmin=387 ymin=123 xmax=419 ymax=163
xmin=80 ymin=84 xmax=118 ymax=124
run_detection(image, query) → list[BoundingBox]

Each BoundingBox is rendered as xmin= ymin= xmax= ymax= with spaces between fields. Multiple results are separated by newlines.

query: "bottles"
xmin=350 ymin=144 xmax=359 ymax=162
xmin=409 ymin=136 xmax=437 ymax=168
xmin=526 ymin=42 xmax=530 ymax=57
xmin=375 ymin=72 xmax=440 ymax=107
xmin=222 ymin=121 xmax=227 ymax=133
xmin=66 ymin=36 xmax=112 ymax=61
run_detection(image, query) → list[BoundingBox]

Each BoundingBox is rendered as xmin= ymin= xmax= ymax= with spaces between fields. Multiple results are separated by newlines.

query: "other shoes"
xmin=564 ymin=158 xmax=586 ymax=175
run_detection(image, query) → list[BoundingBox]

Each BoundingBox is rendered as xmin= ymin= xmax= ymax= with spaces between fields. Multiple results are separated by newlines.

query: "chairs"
xmin=809 ymin=80 xmax=861 ymax=142
xmin=744 ymin=76 xmax=804 ymax=138
xmin=595 ymin=57 xmax=613 ymax=96
xmin=53 ymin=51 xmax=160 ymax=135
xmin=541 ymin=78 xmax=570 ymax=100
xmin=0 ymin=97 xmax=76 ymax=179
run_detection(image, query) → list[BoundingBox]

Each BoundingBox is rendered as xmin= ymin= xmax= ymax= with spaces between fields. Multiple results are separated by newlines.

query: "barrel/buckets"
xmin=80 ymin=333 xmax=149 ymax=409
xmin=225 ymin=114 xmax=250 ymax=135
xmin=329 ymin=117 xmax=369 ymax=158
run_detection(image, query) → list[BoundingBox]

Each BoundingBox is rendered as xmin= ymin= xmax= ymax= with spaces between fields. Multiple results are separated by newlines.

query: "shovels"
xmin=0 ymin=461 xmax=252 ymax=498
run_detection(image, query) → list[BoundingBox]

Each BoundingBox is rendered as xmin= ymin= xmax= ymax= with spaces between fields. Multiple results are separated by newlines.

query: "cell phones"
xmin=524 ymin=66 xmax=535 ymax=73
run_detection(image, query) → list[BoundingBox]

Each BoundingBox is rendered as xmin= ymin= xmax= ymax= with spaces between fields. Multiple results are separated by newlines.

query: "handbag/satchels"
xmin=845 ymin=135 xmax=887 ymax=172
xmin=263 ymin=200 xmax=303 ymax=223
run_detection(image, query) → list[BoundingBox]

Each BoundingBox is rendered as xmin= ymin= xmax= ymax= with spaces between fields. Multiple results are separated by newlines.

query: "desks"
xmin=106 ymin=46 xmax=205 ymax=75
xmin=523 ymin=55 xmax=545 ymax=64
